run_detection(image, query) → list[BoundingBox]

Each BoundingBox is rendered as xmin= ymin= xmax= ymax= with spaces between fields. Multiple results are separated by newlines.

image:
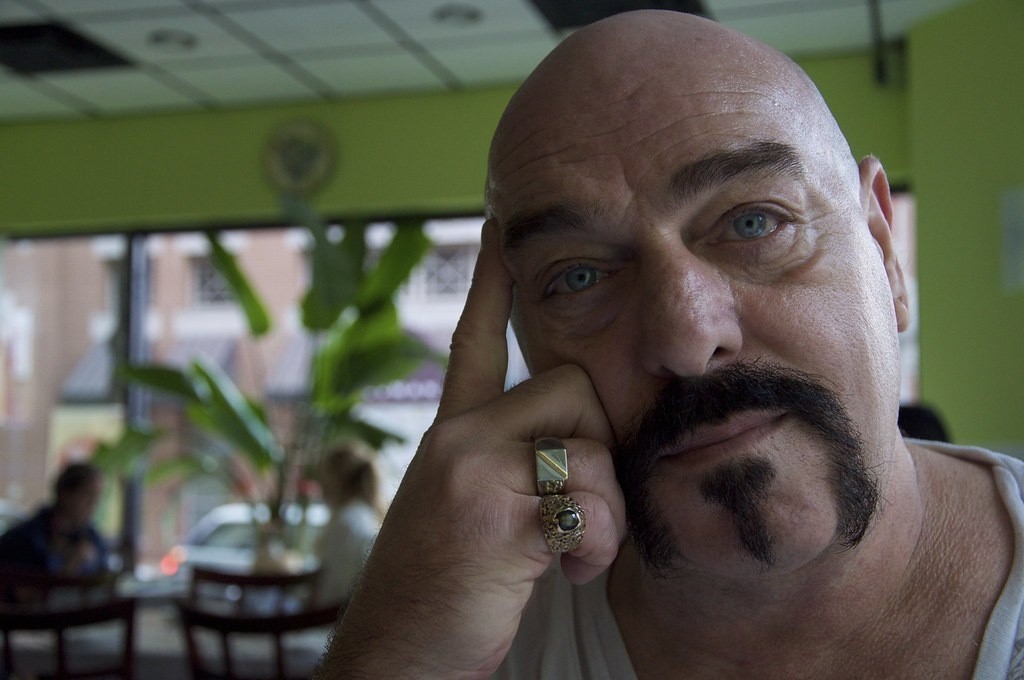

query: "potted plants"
xmin=87 ymin=196 xmax=453 ymax=578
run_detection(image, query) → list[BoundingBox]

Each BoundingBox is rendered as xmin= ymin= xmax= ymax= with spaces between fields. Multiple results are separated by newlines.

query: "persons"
xmin=325 ymin=9 xmax=1024 ymax=680
xmin=0 ymin=465 xmax=110 ymax=625
xmin=316 ymin=444 xmax=383 ymax=608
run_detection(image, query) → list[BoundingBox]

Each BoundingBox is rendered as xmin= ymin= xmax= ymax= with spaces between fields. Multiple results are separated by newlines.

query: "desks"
xmin=182 ymin=583 xmax=348 ymax=680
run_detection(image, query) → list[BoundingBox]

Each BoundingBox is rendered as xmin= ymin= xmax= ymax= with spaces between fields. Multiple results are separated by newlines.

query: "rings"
xmin=539 ymin=495 xmax=586 ymax=554
xmin=535 ymin=438 xmax=567 ymax=495
